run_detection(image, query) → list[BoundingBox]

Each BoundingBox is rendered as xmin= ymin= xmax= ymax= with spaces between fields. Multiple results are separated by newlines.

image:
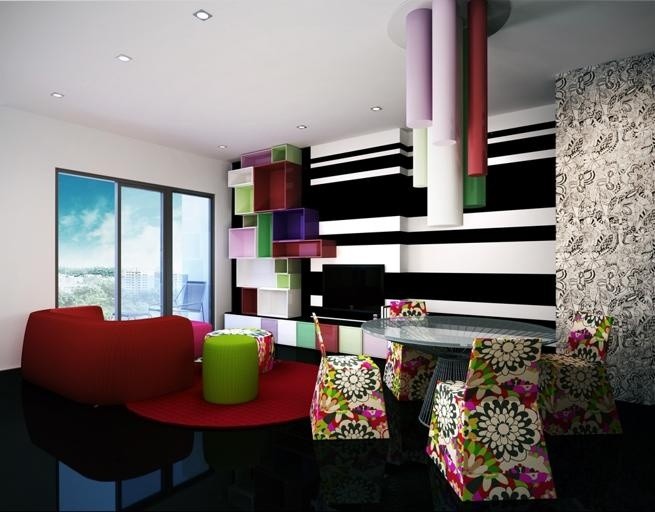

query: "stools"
xmin=202 ymin=333 xmax=259 ymax=405
xmin=189 ymin=319 xmax=213 ymax=361
xmin=207 ymin=329 xmax=275 ymax=375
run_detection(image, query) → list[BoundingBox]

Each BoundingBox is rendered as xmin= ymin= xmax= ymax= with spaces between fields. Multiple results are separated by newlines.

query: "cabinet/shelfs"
xmin=223 ymin=311 xmax=389 ymax=371
xmin=228 ymin=144 xmax=336 ymax=320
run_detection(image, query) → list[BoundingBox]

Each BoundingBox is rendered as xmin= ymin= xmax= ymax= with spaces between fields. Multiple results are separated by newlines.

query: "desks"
xmin=361 ymin=316 xmax=562 ymax=429
xmin=113 ymin=310 xmax=151 ymax=319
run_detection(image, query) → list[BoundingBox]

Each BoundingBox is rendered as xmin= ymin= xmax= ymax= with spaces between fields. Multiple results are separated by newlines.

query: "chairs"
xmin=309 ymin=312 xmax=391 ymax=441
xmin=149 ymin=281 xmax=206 ymax=322
xmin=536 ymin=310 xmax=623 ymax=435
xmin=426 ymin=338 xmax=557 ymax=502
xmin=382 ymin=300 xmax=437 ymax=401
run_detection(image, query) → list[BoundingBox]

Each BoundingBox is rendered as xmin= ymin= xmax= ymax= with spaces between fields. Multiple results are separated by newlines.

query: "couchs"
xmin=20 ymin=304 xmax=195 ymax=402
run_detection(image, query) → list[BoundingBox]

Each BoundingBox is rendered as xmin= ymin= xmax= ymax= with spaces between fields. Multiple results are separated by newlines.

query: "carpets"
xmin=121 ymin=357 xmax=319 ymax=429
